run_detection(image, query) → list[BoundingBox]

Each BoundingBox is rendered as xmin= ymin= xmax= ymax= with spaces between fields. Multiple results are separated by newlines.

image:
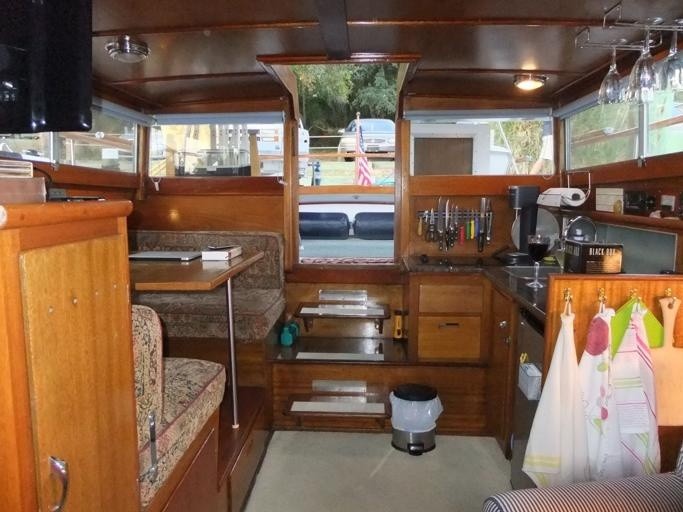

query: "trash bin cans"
xmin=389 ymin=383 xmax=435 ymax=455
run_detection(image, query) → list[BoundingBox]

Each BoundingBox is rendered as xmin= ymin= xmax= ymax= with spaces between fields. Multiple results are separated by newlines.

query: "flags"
xmin=357 ymin=124 xmax=372 ymax=186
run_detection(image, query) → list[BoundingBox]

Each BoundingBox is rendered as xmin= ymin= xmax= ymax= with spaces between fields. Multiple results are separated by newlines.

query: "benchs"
xmin=129 ymin=229 xmax=285 ymax=388
xmin=131 ymin=303 xmax=226 ymax=512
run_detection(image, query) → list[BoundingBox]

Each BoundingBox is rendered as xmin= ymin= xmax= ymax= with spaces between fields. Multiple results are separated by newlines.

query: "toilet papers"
xmin=542 ymin=188 xmax=587 ymax=207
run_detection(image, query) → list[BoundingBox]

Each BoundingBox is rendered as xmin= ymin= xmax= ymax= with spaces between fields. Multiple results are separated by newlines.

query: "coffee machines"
xmin=505 ymin=187 xmax=539 ymax=265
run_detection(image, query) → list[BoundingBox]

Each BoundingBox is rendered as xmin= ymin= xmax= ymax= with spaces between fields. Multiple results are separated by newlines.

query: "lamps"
xmin=104 ymin=34 xmax=151 ymax=64
xmin=513 ymin=75 xmax=549 ymax=91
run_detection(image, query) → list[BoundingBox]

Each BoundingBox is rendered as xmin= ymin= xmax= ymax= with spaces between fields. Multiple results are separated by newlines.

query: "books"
xmin=0 ymin=159 xmax=34 ymax=179
xmin=201 ymin=246 xmax=242 ymax=261
xmin=202 ymin=255 xmax=243 ymax=270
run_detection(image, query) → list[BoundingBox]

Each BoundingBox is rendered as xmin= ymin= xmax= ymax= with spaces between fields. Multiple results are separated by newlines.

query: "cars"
xmin=117 ymin=111 xmax=310 ymax=179
xmin=336 ymin=117 xmax=396 ymax=161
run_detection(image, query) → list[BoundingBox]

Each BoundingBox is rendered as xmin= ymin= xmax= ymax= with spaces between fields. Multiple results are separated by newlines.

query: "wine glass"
xmin=527 ymin=234 xmax=549 ymax=289
xmin=599 ymin=21 xmax=682 ymax=103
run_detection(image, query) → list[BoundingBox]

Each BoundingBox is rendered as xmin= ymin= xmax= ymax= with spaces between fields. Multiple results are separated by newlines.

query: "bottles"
xmin=304 ymin=163 xmax=320 ymax=186
xmin=543 ymin=230 xmax=566 ymax=268
xmin=613 ymin=191 xmax=683 ymax=218
xmin=392 ymin=310 xmax=403 ymax=341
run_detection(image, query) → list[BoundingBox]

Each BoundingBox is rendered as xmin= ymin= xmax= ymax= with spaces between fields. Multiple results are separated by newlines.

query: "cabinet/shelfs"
xmin=407 ymin=271 xmax=518 ymax=460
xmin=1 ymin=199 xmax=142 ymax=512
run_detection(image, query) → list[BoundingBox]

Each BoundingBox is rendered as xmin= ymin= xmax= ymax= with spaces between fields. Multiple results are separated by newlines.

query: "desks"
xmin=129 ymin=248 xmax=265 ymax=429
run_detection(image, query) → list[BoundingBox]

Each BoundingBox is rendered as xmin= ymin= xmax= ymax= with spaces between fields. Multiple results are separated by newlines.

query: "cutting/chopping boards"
xmin=647 ymin=298 xmax=682 ymax=426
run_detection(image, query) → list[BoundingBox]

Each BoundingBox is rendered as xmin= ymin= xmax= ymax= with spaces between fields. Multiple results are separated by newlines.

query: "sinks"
xmin=501 ymin=265 xmax=569 ymax=281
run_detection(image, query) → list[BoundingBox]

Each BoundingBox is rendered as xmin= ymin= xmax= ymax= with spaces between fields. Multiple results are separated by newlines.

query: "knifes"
xmin=415 ymin=196 xmax=492 ymax=253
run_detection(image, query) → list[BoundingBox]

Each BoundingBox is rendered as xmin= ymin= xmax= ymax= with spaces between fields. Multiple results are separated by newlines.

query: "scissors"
xmin=425 ymin=208 xmax=439 ymax=242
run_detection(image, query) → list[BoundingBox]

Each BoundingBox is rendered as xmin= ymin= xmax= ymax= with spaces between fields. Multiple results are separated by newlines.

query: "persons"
xmin=529 ymin=121 xmax=554 ymax=175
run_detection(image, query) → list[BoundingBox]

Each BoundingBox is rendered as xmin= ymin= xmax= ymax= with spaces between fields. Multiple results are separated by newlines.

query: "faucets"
xmin=524 ymin=260 xmax=545 ymax=289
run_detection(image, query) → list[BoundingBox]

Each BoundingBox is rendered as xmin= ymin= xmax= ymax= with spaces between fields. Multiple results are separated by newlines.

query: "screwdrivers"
xmin=459 ymin=206 xmax=478 ymax=246
